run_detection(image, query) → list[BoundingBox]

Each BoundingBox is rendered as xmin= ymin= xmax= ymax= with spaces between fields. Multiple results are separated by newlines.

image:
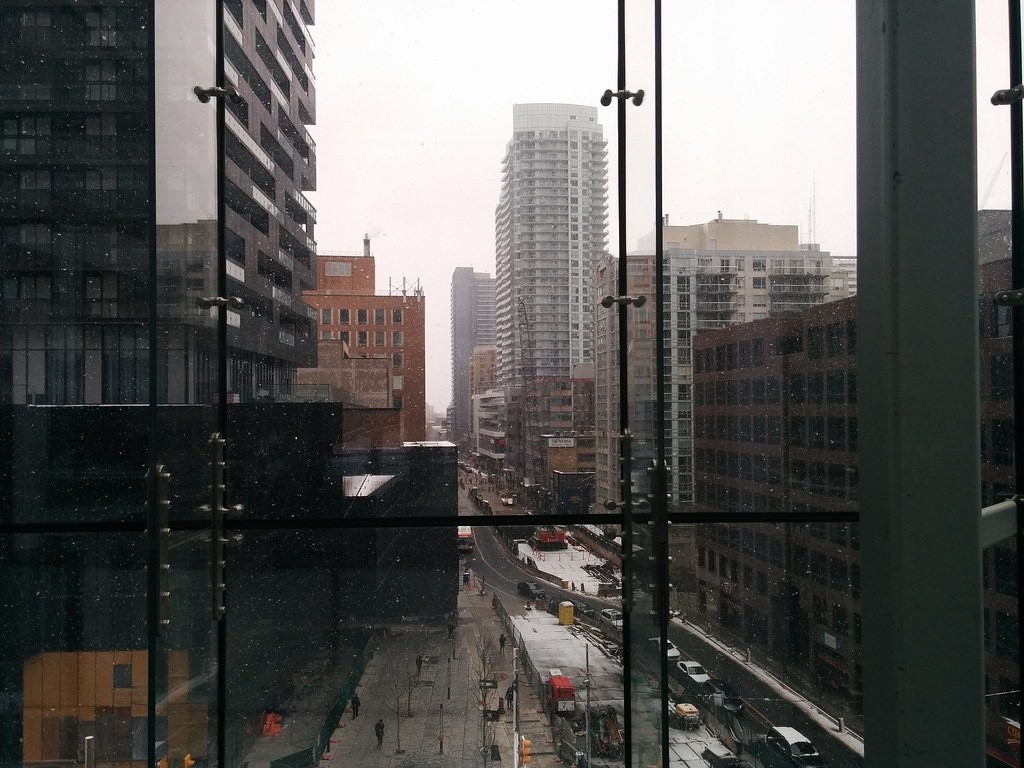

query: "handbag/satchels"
xmin=380 ymin=732 xmax=384 ymax=736
xmin=351 ymin=704 xmax=354 ymax=708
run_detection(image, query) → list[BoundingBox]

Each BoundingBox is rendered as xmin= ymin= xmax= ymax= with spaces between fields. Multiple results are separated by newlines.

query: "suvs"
xmin=597 ymin=608 xmax=623 ymax=632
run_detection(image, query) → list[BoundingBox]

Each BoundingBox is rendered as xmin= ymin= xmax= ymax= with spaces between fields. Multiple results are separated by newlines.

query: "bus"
xmin=458 ymin=526 xmax=476 ymax=553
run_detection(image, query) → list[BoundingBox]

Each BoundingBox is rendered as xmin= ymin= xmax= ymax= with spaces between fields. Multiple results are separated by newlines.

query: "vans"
xmin=763 ymin=725 xmax=825 ymax=768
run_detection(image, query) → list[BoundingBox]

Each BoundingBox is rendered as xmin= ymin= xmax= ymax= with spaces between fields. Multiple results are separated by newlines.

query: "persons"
xmin=505 ymin=687 xmax=513 ymax=710
xmin=448 ymin=622 xmax=454 ymax=642
xmin=351 ymin=693 xmax=360 ymax=720
xmin=375 ymin=719 xmax=385 ymax=748
xmin=499 ymin=634 xmax=507 ymax=655
xmin=416 ymin=655 xmax=422 ymax=673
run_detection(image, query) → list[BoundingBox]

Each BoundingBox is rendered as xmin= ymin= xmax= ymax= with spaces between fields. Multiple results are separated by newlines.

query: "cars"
xmin=568 ymin=597 xmax=595 ymax=617
xmin=676 ymin=659 xmax=711 ymax=686
xmin=703 ymin=678 xmax=745 ymax=715
xmin=647 ymin=636 xmax=681 ymax=659
xmin=516 ymin=580 xmax=547 ymax=601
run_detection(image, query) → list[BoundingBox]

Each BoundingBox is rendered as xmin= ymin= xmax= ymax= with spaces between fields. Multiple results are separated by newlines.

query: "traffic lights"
xmin=183 ymin=753 xmax=196 ymax=768
xmin=518 ymin=735 xmax=533 ymax=768
xmin=156 ymin=756 xmax=168 ymax=768
